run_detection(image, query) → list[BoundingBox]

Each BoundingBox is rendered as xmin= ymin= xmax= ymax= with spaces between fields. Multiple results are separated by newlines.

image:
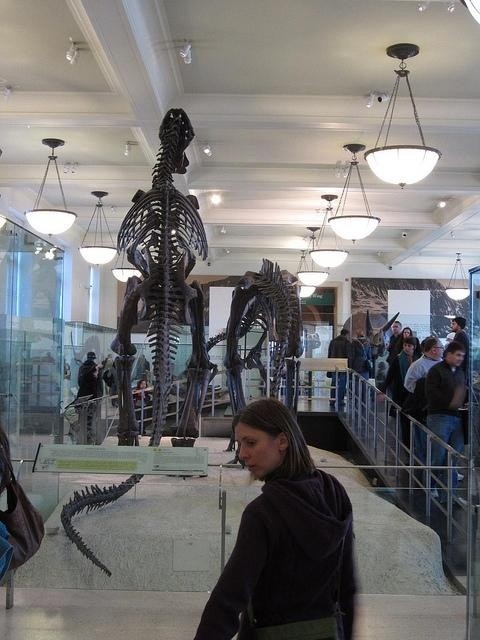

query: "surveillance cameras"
xmin=378 ymin=91 xmax=392 ymax=102
xmin=401 ymin=232 xmax=407 ymax=238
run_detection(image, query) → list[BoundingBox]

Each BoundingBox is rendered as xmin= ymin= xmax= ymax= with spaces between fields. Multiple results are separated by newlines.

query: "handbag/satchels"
xmin=1 ymin=481 xmax=44 ymax=570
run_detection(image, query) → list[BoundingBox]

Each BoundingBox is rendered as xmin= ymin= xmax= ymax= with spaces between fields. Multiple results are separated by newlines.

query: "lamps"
xmin=111 ymin=248 xmax=142 ymax=283
xmin=444 ymin=249 xmax=470 ymax=302
xmin=179 ymin=43 xmax=192 ymax=64
xmin=78 ymin=191 xmax=117 ymax=265
xmin=294 ymin=44 xmax=440 ymax=298
xmin=63 ymin=162 xmax=78 ymax=174
xmin=24 ymin=138 xmax=76 ymax=237
xmin=201 ymin=143 xmax=212 ymax=157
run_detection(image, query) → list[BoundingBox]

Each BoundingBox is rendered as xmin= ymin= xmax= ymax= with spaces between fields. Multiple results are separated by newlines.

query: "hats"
xmin=454 ymin=317 xmax=466 ymax=329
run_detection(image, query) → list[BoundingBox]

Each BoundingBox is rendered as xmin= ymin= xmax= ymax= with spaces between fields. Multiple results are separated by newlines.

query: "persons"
xmin=77 ymin=352 xmax=151 ymax=435
xmin=0 ymin=426 xmax=45 ymax=590
xmin=326 ymin=316 xmax=470 ymax=504
xmin=192 ymin=398 xmax=358 ymax=640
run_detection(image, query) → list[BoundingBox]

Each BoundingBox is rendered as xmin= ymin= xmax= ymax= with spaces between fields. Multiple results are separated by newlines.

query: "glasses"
xmin=433 ymin=346 xmax=444 ymax=350
